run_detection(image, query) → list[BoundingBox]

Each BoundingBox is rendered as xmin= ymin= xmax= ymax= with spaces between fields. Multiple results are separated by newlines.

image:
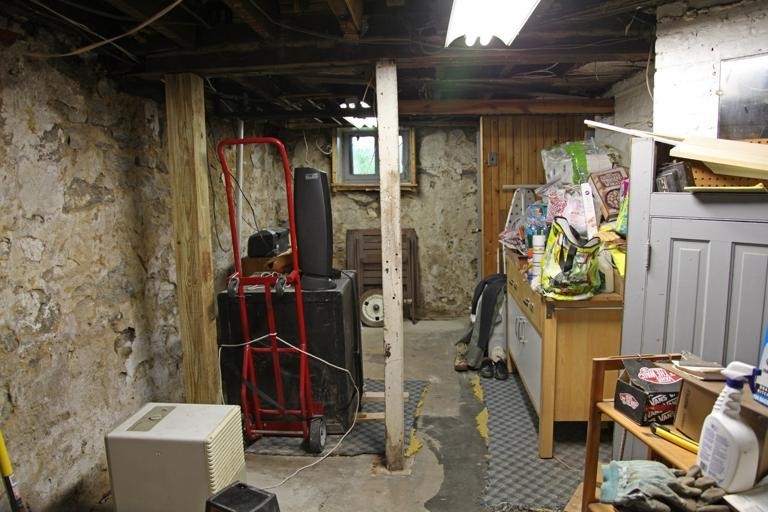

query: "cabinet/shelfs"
xmin=612 ymin=129 xmax=768 ymax=460
xmin=577 ymin=353 xmax=768 ymax=512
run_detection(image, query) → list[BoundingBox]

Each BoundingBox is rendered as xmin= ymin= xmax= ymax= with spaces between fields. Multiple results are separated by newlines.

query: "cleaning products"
xmin=694 ymin=361 xmax=762 ymax=495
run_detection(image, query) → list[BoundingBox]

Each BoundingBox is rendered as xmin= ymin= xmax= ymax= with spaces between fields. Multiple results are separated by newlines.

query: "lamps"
xmin=439 ymin=0 xmax=547 ymax=51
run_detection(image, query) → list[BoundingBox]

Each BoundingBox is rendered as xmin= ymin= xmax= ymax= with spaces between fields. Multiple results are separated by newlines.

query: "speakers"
xmin=295 ymin=167 xmax=336 ymax=290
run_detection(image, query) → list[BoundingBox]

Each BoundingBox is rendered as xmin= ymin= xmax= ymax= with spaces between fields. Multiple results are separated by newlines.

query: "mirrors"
xmin=715 ymin=50 xmax=768 ymax=143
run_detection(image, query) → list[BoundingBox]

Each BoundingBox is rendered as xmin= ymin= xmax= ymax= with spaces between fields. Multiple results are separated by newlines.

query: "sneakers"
xmin=494 ymin=358 xmax=509 ymax=381
xmin=480 ymin=358 xmax=494 ymax=378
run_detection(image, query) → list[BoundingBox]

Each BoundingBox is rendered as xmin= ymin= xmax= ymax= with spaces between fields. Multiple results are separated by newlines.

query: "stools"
xmin=204 ymin=479 xmax=281 ymax=510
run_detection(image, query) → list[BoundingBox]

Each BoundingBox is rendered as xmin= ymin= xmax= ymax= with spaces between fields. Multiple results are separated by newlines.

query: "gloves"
xmin=599 ymin=459 xmax=730 ymax=512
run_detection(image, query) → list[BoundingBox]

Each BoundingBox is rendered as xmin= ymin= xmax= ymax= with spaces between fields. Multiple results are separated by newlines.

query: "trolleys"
xmin=217 ymin=136 xmax=329 ymax=459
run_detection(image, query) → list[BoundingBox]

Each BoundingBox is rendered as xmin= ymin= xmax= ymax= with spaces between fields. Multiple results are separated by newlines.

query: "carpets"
xmin=476 ymin=370 xmax=615 ymax=511
xmin=234 ymin=378 xmax=428 ymax=456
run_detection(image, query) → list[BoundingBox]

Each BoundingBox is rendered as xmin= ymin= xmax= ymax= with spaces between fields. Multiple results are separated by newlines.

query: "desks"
xmin=503 ymin=235 xmax=627 ymax=459
xmin=217 ymin=267 xmax=363 ymax=438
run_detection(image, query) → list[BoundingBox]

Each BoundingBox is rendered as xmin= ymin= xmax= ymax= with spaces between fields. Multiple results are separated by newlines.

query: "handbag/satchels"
xmin=538 ymin=215 xmax=603 ymax=302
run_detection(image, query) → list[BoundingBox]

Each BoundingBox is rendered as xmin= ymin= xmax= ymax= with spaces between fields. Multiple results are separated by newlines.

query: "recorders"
xmin=248 ymin=228 xmax=289 ymax=257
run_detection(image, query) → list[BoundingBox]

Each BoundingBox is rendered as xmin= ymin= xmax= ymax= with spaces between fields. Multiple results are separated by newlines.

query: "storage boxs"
xmin=673 ymin=377 xmax=767 ymax=483
xmin=549 ymin=155 xmax=612 ymax=185
